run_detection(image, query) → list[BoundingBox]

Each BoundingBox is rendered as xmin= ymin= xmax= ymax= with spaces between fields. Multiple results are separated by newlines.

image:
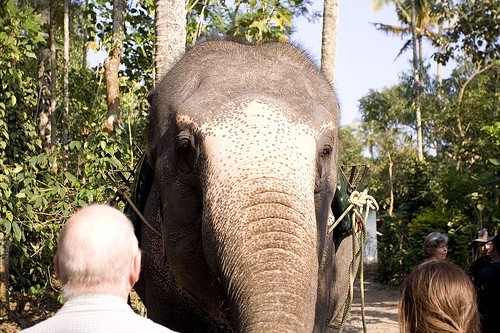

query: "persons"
xmin=423 ymin=232 xmax=449 ymax=261
xmin=18 ymin=203 xmax=181 ymax=333
xmin=467 ymin=227 xmax=500 ymax=333
xmin=399 ymin=259 xmax=483 ymax=333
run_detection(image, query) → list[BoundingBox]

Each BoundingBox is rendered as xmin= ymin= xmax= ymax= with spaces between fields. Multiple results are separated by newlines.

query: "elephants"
xmin=121 ymin=27 xmax=362 ymax=333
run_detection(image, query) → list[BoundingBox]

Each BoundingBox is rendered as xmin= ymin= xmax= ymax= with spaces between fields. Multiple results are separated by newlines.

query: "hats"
xmin=469 ymin=229 xmax=496 ymax=248
